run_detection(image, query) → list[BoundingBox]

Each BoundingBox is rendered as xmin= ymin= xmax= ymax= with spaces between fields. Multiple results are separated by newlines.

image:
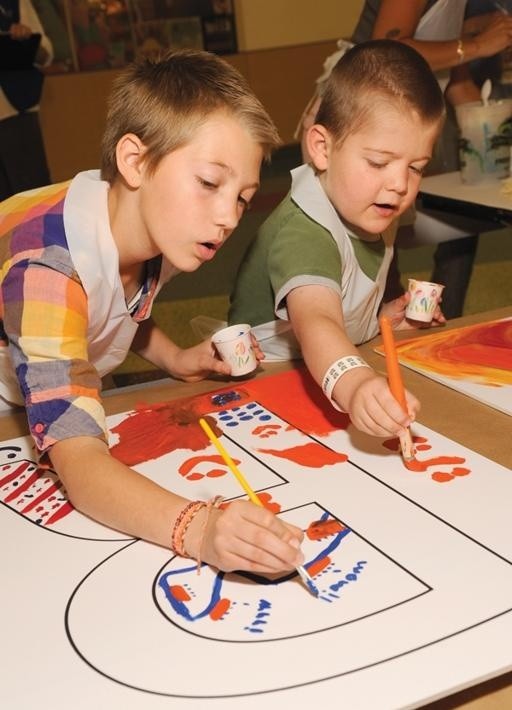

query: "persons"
xmin=227 ymin=36 xmax=449 ymax=439
xmin=0 ymin=0 xmax=55 ymax=195
xmin=373 ymin=1 xmax=512 ymax=322
xmin=0 ymin=48 xmax=305 ymax=578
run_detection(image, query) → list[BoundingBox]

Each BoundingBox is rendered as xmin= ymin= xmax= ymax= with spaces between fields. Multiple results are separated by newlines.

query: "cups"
xmin=407 ymin=280 xmax=445 ymax=327
xmin=213 ymin=325 xmax=259 ymax=376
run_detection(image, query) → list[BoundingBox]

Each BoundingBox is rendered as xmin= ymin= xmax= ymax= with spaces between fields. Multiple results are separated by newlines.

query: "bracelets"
xmin=455 ymin=37 xmax=464 ymax=63
xmin=322 ymin=355 xmax=372 ymax=413
xmin=171 ymin=495 xmax=206 ymax=558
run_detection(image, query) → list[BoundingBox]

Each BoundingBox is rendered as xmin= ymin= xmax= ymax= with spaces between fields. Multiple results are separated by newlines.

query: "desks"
xmin=1 ymin=308 xmax=509 ymax=708
xmin=38 ymin=37 xmax=355 ymax=183
xmin=416 ymin=168 xmax=511 ymax=316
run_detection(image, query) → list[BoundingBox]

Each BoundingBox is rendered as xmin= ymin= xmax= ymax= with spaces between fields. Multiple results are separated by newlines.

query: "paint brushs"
xmin=378 ymin=315 xmax=428 ymax=471
xmin=198 ymin=418 xmax=318 ymax=597
